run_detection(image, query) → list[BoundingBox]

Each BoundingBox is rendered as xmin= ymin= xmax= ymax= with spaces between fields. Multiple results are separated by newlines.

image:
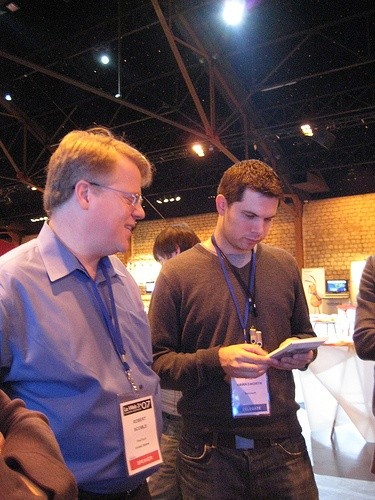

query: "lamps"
xmin=156 ymin=193 xmax=181 ymax=204
xmin=31 ymin=215 xmax=48 ymax=222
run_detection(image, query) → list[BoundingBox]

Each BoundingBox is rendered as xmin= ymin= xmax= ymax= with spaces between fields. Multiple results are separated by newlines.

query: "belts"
xmin=79 ymin=484 xmax=142 ymax=500
xmin=202 ymin=432 xmax=290 ymax=449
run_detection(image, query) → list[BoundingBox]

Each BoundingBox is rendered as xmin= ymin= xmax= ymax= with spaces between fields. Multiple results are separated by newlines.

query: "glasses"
xmin=71 ymin=181 xmax=144 ymax=206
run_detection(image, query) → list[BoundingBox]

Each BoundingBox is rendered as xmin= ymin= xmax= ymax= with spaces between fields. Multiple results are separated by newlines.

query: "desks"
xmin=307 ymin=335 xmax=375 ymax=443
xmin=311 ymin=319 xmax=336 ymax=333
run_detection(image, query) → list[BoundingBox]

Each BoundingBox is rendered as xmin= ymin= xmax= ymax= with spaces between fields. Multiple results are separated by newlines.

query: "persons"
xmin=147 ymin=224 xmax=203 ymax=500
xmin=149 ymin=159 xmax=319 ymax=500
xmin=0 ymin=127 xmax=162 ymax=500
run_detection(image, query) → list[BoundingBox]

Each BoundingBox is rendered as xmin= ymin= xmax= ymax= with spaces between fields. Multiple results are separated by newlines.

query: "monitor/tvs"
xmin=326 ymin=279 xmax=348 ymax=293
xmin=146 ymin=282 xmax=154 ymax=293
